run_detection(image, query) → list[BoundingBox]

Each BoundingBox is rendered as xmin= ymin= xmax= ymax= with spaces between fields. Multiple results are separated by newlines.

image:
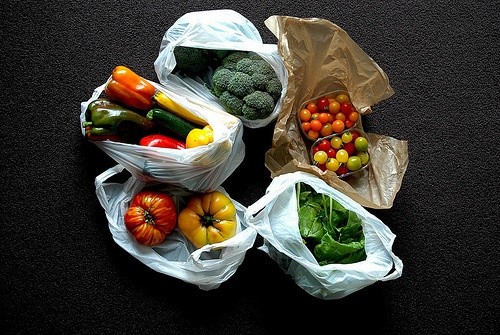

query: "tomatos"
xmin=300 ymin=93 xmax=370 ymax=174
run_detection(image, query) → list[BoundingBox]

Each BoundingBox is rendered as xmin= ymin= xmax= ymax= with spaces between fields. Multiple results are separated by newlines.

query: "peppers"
xmin=177 ymin=191 xmax=238 ymax=249
xmin=81 ymin=66 xmax=213 ymax=148
xmin=124 ymin=193 xmax=178 ymax=246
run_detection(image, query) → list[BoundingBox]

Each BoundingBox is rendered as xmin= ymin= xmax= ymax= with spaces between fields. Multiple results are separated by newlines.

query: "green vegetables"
xmin=297 ymin=181 xmax=367 ymax=266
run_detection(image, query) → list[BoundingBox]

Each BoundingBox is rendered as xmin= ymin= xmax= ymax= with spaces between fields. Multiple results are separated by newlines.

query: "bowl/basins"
xmin=297 ymin=89 xmax=372 ymax=180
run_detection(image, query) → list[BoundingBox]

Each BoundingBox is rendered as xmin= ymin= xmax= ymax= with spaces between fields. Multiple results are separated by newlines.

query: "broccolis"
xmin=171 ymin=46 xmax=282 ymax=120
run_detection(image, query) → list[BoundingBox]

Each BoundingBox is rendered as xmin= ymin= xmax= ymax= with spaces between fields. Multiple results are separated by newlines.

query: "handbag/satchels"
xmin=94 ymin=163 xmax=258 ymax=291
xmin=78 ymin=75 xmax=246 ymax=194
xmin=245 ymin=170 xmax=404 ymax=301
xmin=154 ymin=8 xmax=289 ymax=130
